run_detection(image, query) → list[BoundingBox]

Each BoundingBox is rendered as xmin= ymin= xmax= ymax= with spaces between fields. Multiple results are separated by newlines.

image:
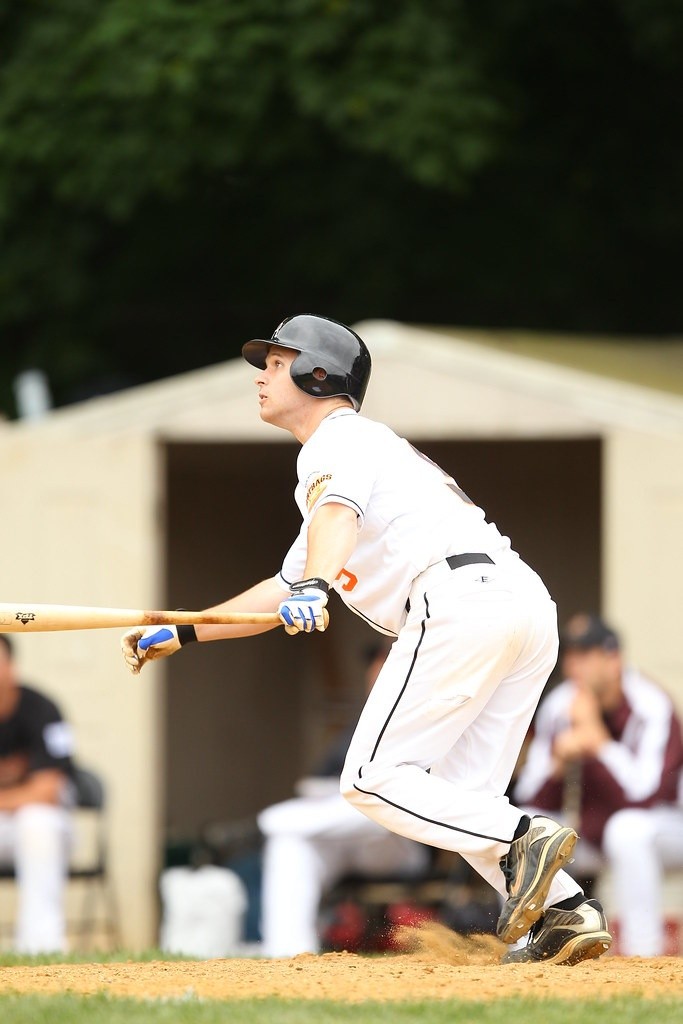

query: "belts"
xmin=404 ymin=553 xmax=496 ymax=613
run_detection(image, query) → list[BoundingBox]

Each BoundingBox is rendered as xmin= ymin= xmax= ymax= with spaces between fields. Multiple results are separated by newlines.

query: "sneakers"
xmin=496 ymin=815 xmax=578 ymax=944
xmin=501 ymin=899 xmax=612 ymax=966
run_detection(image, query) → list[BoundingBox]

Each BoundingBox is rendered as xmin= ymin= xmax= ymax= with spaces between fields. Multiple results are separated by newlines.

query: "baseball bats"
xmin=0 ymin=601 xmax=330 ymax=634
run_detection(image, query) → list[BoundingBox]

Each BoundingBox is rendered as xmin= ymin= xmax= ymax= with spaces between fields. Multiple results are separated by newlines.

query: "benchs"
xmin=341 ymin=871 xmax=599 ymax=900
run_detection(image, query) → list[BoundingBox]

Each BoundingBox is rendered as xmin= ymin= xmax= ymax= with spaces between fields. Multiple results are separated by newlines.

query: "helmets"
xmin=242 ymin=313 xmax=372 ymax=413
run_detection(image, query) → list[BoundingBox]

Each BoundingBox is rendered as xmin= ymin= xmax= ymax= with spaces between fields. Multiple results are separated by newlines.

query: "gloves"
xmin=277 ymin=577 xmax=333 ymax=635
xmin=121 ymin=625 xmax=198 ymax=675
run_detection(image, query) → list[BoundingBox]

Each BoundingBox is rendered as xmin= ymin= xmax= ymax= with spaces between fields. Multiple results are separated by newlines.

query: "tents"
xmin=0 ymin=320 xmax=683 ymax=953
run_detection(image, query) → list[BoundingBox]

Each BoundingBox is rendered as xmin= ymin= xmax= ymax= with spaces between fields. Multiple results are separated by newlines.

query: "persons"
xmin=0 ymin=613 xmax=683 ymax=960
xmin=121 ymin=315 xmax=611 ymax=970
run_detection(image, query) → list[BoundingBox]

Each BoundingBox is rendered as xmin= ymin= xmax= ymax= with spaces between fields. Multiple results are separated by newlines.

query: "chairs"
xmin=0 ymin=771 xmax=123 ymax=957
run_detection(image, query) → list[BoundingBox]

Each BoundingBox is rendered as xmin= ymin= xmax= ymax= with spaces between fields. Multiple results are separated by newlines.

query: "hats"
xmin=558 ymin=615 xmax=617 ymax=653
